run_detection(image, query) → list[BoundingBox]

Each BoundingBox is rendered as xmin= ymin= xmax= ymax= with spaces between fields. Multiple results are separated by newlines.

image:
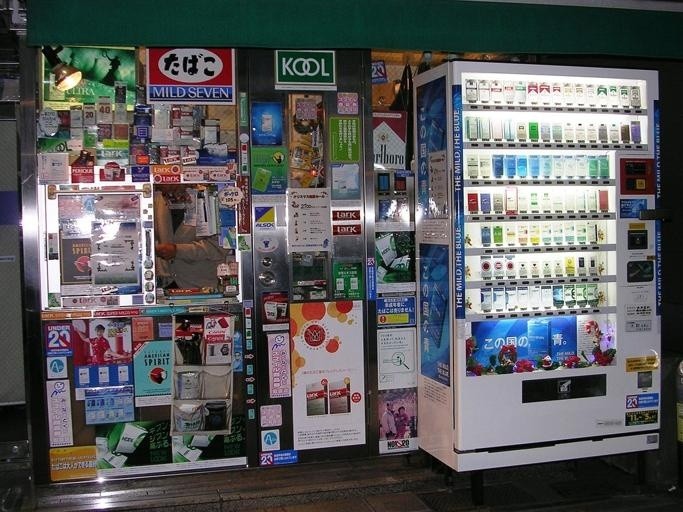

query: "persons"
xmin=75 ymin=323 xmax=129 ymax=365
xmin=153 ymin=185 xmax=237 ymax=297
xmin=394 ymin=406 xmax=409 ymax=438
xmin=380 ymin=400 xmax=397 ymax=439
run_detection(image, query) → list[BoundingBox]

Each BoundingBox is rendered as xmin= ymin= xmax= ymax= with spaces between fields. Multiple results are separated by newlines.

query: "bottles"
xmin=531 ymin=263 xmax=539 ymax=277
xmin=589 ymin=260 xmax=597 ymax=274
xmin=576 ymin=257 xmax=586 ymax=274
xmin=564 ymin=122 xmax=574 ymax=142
xmin=599 ymin=121 xmax=608 ymax=143
xmin=519 ymin=263 xmax=528 ymax=278
xmin=542 ymin=192 xmax=552 ymax=212
xmin=542 ymin=263 xmax=551 ymax=277
xmin=610 ymin=123 xmax=620 ymax=143
xmin=576 ymin=122 xmax=586 ymax=143
xmin=554 ymin=263 xmax=563 ymax=277
xmin=588 ymin=122 xmax=598 ymax=142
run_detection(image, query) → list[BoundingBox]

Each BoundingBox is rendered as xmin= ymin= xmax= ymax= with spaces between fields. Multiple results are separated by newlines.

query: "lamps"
xmin=42 ymin=44 xmax=84 ymax=93
